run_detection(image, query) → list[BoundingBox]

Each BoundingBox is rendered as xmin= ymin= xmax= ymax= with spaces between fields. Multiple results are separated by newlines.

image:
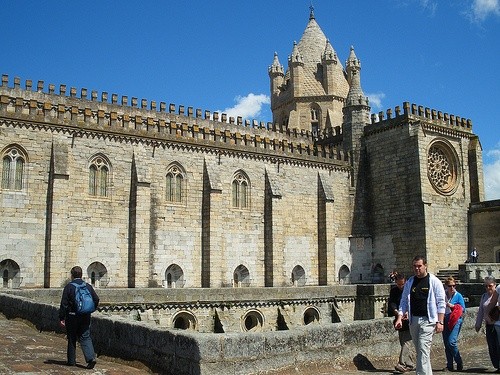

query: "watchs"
xmin=436 ymin=320 xmax=444 ymax=325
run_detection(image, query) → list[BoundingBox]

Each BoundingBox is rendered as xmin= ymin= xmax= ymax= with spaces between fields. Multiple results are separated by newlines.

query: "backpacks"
xmin=75 ymin=286 xmax=95 ymax=313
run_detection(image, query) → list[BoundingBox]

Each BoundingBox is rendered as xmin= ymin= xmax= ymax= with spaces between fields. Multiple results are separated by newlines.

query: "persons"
xmin=442 ymin=278 xmax=466 ymax=372
xmin=476 ymin=269 xmax=500 ymax=373
xmin=387 ymin=271 xmax=398 ymax=283
xmin=471 ymin=248 xmax=477 ymax=263
xmin=59 ymin=266 xmax=99 ymax=369
xmin=395 ymin=256 xmax=447 ymax=375
xmin=387 ymin=274 xmax=416 ymax=372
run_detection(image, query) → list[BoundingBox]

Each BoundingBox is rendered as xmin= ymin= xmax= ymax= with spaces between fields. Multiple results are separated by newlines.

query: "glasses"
xmin=485 ymin=285 xmax=492 ymax=287
xmin=448 ymin=284 xmax=457 ymax=288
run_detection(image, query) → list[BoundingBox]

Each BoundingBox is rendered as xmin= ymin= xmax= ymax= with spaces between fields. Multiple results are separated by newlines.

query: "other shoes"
xmin=394 ymin=363 xmax=414 ymax=373
xmin=66 ymin=362 xmax=74 ymax=365
xmin=86 ymin=361 xmax=96 ymax=369
